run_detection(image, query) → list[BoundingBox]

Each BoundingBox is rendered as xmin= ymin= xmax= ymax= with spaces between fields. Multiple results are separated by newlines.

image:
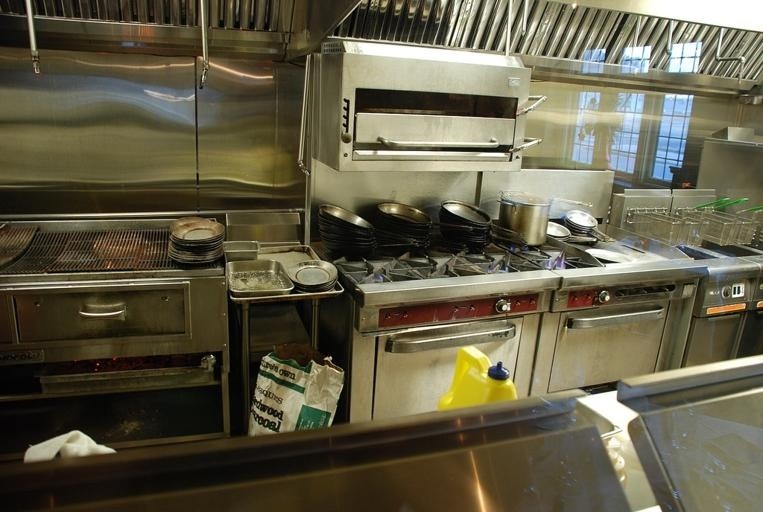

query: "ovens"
xmin=322 ymin=41 xmax=532 ymax=172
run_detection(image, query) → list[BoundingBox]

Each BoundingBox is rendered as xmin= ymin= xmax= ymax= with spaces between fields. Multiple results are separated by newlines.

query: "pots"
xmin=317 ymin=191 xmax=610 ymax=259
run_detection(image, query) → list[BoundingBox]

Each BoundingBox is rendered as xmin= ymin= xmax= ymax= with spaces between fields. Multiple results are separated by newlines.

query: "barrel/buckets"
xmin=440 ymin=344 xmax=518 ymax=414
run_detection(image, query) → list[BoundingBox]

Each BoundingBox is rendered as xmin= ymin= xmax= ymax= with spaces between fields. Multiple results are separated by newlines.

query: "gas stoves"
xmin=317 ymin=241 xmax=604 ymax=288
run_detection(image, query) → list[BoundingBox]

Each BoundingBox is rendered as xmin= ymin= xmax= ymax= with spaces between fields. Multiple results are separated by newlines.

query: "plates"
xmin=288 ymin=260 xmax=339 ymax=293
xmin=168 ymin=217 xmax=226 ymax=264
